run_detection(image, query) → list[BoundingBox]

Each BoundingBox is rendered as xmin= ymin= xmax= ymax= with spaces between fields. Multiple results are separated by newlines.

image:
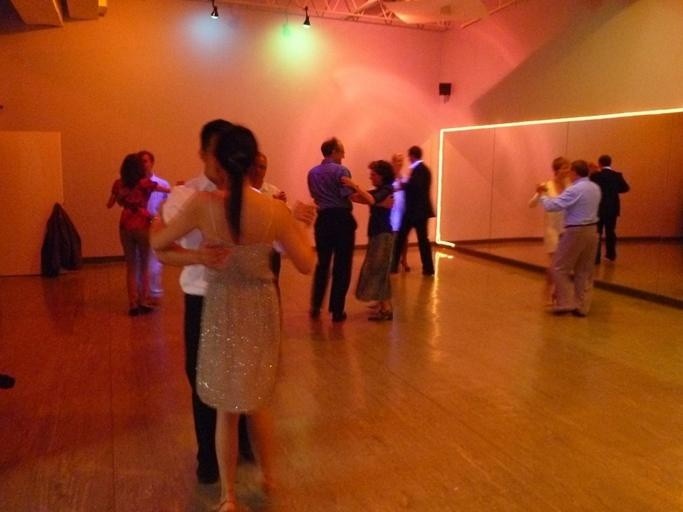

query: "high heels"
xmin=400 ymin=261 xmax=410 ymax=272
xmin=368 ymin=311 xmax=393 ymax=321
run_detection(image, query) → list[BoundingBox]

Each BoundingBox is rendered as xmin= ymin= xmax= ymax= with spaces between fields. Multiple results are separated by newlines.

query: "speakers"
xmin=438 ymin=82 xmax=451 ymax=96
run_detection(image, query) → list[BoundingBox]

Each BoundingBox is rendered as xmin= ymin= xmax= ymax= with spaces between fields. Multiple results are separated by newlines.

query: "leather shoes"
xmin=332 ymin=313 xmax=346 ymax=322
xmin=197 ymin=457 xmax=220 ymax=484
xmin=129 ymin=304 xmax=153 ymax=315
xmin=0 ymin=374 xmax=14 ymax=388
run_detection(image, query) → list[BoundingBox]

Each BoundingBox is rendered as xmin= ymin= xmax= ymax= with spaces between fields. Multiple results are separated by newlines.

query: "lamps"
xmin=203 ymin=0 xmax=313 ymax=28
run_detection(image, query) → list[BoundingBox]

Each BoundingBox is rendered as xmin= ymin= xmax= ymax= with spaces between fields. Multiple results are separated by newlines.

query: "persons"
xmin=527 ymin=156 xmax=574 ymax=311
xmin=587 ymin=161 xmax=600 ymax=179
xmin=107 ymin=150 xmax=184 ymax=314
xmin=256 ymin=153 xmax=287 ymax=285
xmin=306 ymin=137 xmax=437 ymax=320
xmin=535 ymin=159 xmax=604 ymax=319
xmin=588 ymin=155 xmax=631 ymax=265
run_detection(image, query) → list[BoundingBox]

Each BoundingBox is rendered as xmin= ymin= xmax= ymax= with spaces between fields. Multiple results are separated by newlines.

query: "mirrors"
xmin=434 ymin=111 xmax=681 ymax=307
